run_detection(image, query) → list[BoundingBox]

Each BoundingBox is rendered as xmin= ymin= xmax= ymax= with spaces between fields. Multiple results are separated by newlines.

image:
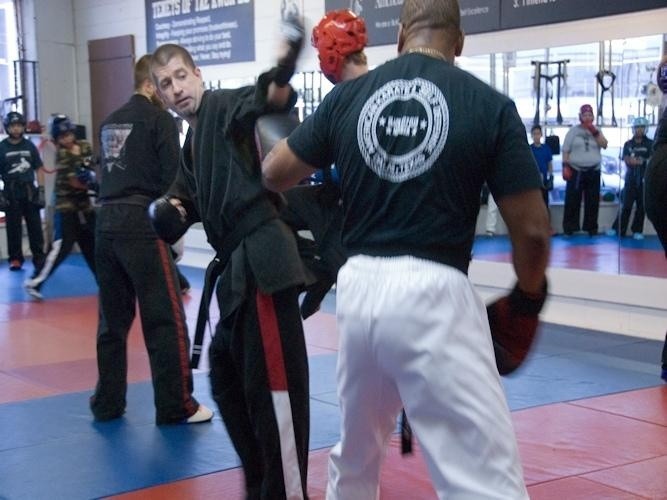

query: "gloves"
xmin=561 ymin=162 xmax=573 ymax=181
xmin=0 ymin=190 xmax=11 ymax=212
xmin=583 ymin=120 xmax=600 ymax=137
xmin=150 ymin=191 xmax=190 ymax=246
xmin=33 ymin=187 xmax=47 ymax=208
xmin=483 ymin=278 xmax=551 ymax=376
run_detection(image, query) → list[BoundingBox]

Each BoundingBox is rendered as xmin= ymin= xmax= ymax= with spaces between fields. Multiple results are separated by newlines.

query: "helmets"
xmin=631 ymin=117 xmax=649 ymax=136
xmin=53 ymin=115 xmax=75 ymax=146
xmin=579 ymin=104 xmax=594 ymax=123
xmin=4 ymin=111 xmax=26 ymax=138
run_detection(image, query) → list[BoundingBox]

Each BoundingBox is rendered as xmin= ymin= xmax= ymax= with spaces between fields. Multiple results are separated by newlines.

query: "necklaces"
xmin=408 ymin=48 xmax=446 ymax=61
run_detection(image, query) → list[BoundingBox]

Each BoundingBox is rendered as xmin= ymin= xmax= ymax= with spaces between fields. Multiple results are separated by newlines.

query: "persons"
xmin=148 ymin=13 xmax=318 ymax=500
xmin=258 ymin=0 xmax=551 ymax=500
xmin=528 ymin=103 xmax=655 ymax=240
xmin=88 ymin=51 xmax=216 ymax=427
xmin=0 ymin=111 xmax=47 ymax=271
xmin=486 ymin=193 xmax=498 ymax=237
xmin=22 ymin=114 xmax=100 ymax=300
xmin=641 ymin=106 xmax=667 ymax=381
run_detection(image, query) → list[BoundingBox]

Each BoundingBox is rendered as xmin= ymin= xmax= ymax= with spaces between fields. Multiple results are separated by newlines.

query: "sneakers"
xmin=24 ymin=281 xmax=43 ymax=299
xmin=9 ymin=260 xmax=21 ymax=269
xmin=176 ymin=405 xmax=213 ymax=424
